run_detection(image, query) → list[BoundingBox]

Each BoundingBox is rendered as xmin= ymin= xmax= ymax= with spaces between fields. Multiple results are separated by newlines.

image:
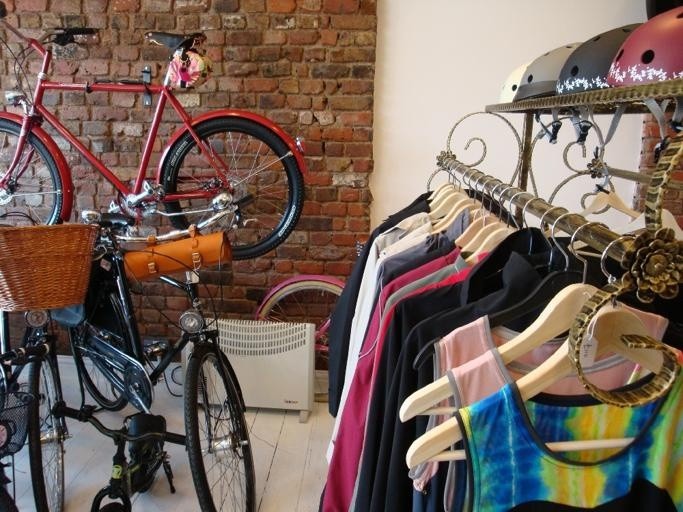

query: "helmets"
xmin=502 ymin=8 xmax=683 ymax=101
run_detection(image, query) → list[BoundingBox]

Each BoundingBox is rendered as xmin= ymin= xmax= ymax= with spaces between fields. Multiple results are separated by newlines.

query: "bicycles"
xmin=254 ymin=275 xmax=346 ymax=404
xmin=67 ymin=208 xmax=259 ymax=507
xmin=0 ymin=211 xmax=94 ymax=505
xmin=1 ymin=1 xmax=313 ymax=260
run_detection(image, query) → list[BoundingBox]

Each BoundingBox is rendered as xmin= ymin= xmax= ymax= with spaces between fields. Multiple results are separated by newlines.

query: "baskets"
xmin=1 ymin=224 xmax=98 ymax=312
xmin=0 ymin=392 xmax=35 ymax=458
